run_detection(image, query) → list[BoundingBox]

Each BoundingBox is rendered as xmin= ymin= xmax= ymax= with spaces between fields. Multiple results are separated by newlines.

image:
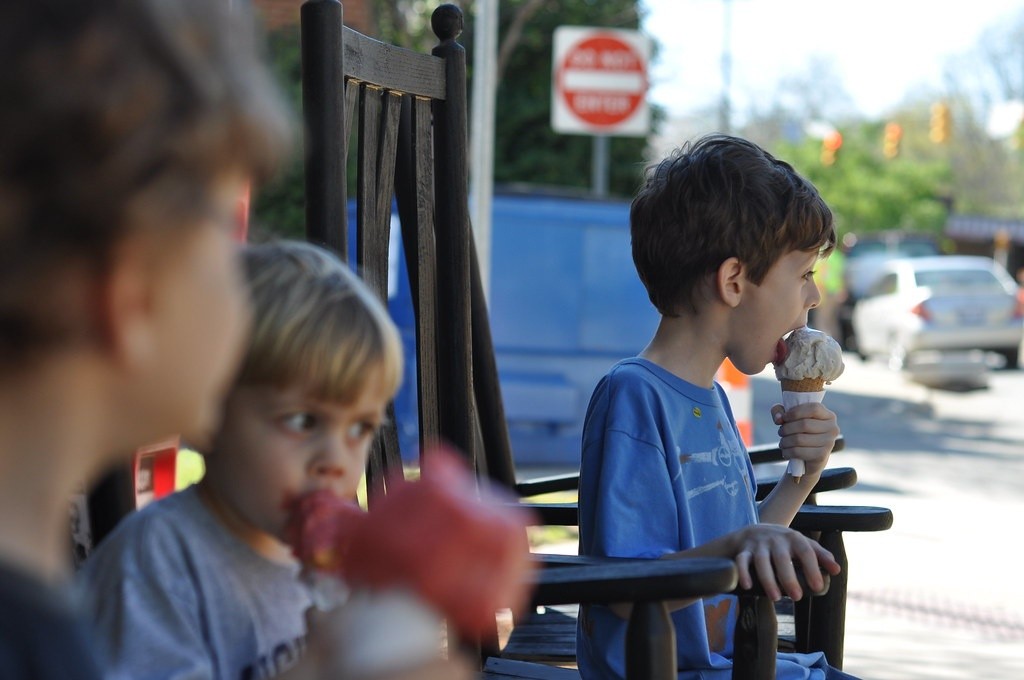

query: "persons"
xmin=78 ymin=237 xmax=404 ymax=680
xmin=0 ymin=0 xmax=475 ymax=680
xmin=578 ymin=134 xmax=861 ymax=680
xmin=809 ymin=246 xmax=848 ymax=338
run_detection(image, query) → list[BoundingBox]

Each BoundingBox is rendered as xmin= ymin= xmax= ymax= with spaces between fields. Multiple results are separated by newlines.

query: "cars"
xmin=854 ymin=251 xmax=1020 ymax=366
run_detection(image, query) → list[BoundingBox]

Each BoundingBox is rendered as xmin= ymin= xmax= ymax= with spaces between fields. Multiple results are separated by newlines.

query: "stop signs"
xmin=548 ymin=26 xmax=650 ymax=135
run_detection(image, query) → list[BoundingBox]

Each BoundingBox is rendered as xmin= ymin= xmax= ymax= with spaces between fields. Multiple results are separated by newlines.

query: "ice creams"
xmin=774 ymin=327 xmax=845 ymax=483
xmin=286 ymin=490 xmax=370 ymax=610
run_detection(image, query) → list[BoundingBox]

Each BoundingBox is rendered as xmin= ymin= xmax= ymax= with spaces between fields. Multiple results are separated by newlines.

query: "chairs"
xmin=300 ymin=1 xmax=893 ymax=680
